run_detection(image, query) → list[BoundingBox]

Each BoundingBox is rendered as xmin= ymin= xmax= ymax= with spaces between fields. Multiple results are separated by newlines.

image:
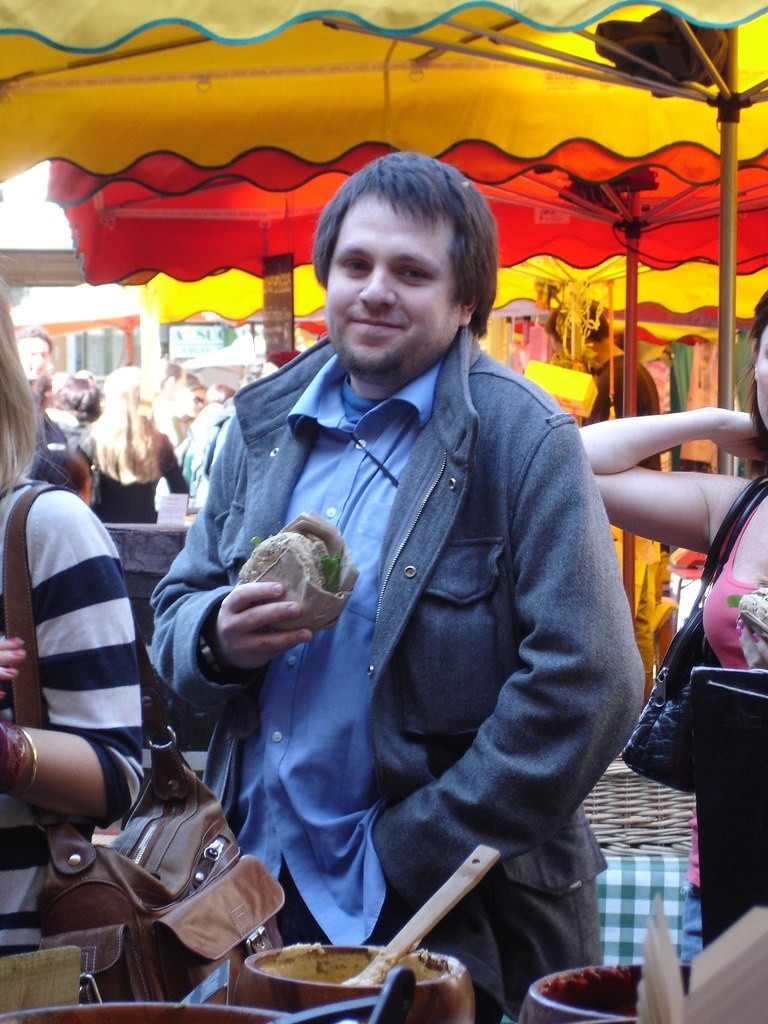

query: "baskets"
xmin=583 ymin=762 xmax=695 ymax=857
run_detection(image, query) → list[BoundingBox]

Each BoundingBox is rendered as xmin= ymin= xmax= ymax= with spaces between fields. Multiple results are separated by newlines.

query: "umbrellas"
xmin=0 ymin=0 xmax=768 ymax=475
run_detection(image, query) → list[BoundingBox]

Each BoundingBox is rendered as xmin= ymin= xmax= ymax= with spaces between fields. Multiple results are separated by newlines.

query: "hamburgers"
xmin=238 ymin=529 xmax=341 ymax=595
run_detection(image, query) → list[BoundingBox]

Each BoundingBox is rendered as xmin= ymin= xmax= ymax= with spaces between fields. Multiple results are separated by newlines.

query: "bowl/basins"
xmin=518 ymin=964 xmax=690 ymax=1023
xmin=231 ymin=943 xmax=477 ymax=1024
xmin=0 ymin=1001 xmax=313 ymax=1024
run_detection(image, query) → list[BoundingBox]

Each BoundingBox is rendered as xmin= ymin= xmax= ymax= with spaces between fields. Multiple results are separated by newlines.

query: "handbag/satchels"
xmin=2 ymin=484 xmax=285 ymax=1003
xmin=622 ymin=475 xmax=768 ymax=793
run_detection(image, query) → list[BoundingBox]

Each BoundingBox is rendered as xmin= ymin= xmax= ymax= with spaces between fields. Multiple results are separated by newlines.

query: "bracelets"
xmin=0 ymin=720 xmax=38 ymax=793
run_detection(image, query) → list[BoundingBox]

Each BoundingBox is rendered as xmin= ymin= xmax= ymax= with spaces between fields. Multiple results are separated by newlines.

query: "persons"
xmin=547 ymin=300 xmax=661 ymax=712
xmin=151 ymin=153 xmax=646 ymax=1024
xmin=0 ymin=277 xmax=144 ymax=958
xmin=17 ymin=326 xmax=302 ymax=527
xmin=578 ymin=291 xmax=767 ymax=962
